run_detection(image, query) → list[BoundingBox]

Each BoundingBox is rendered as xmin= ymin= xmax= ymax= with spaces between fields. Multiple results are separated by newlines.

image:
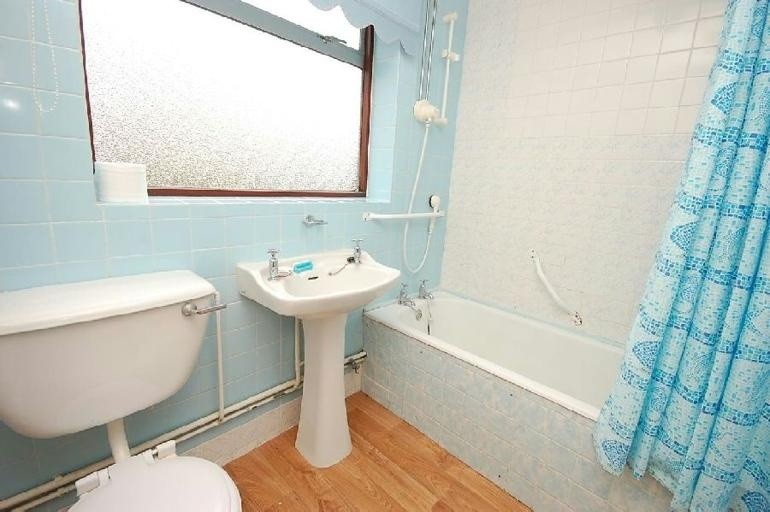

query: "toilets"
xmin=1 ymin=270 xmax=244 ymax=512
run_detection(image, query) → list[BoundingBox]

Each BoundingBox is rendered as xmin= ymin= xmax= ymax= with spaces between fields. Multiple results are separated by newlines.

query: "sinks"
xmin=237 ymin=245 xmax=401 ymax=316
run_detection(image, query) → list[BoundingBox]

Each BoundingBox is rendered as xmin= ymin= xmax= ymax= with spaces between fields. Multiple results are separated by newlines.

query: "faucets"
xmin=399 ymin=283 xmax=416 ymax=307
xmin=346 ymin=237 xmax=363 ymax=263
xmin=420 ymin=279 xmax=435 ymax=301
xmin=266 ymin=249 xmax=292 ymax=279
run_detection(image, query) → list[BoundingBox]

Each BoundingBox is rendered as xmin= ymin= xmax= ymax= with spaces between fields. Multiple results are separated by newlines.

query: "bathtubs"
xmin=365 ymin=291 xmax=623 ymax=419
